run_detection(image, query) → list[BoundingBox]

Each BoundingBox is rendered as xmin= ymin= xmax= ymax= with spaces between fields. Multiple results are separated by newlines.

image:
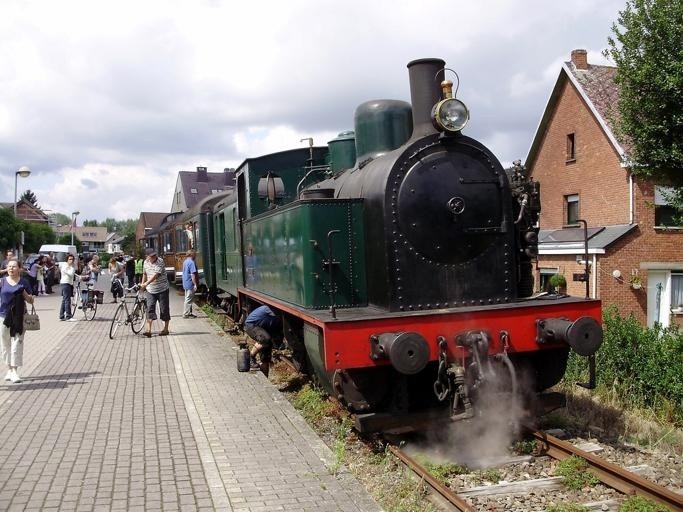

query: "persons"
xmin=0 ymin=250 xmax=15 ymax=278
xmin=26 ymin=250 xmax=145 ymax=304
xmin=244 ymin=242 xmax=258 ymax=290
xmin=0 ymin=258 xmax=34 ymax=384
xmin=58 ymin=253 xmax=77 ymax=322
xmin=243 ymin=304 xmax=281 ymax=380
xmin=181 ymin=249 xmax=198 ymax=319
xmin=139 ymin=247 xmax=171 ymax=337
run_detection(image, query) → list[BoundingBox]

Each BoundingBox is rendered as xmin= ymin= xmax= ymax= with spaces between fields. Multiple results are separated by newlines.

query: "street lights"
xmin=71 ymin=211 xmax=80 ymax=246
xmin=13 ymin=166 xmax=30 ymax=221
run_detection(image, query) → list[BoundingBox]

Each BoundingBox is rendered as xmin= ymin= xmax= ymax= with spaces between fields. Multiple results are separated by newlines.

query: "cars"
xmin=22 ymin=253 xmax=37 ymax=271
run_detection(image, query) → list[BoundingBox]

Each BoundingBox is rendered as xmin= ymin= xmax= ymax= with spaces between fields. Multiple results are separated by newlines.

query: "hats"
xmin=145 ymin=248 xmax=157 ymax=258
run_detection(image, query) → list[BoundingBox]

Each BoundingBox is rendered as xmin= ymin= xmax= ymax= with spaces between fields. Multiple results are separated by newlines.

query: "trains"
xmin=138 ymin=59 xmax=603 ymax=435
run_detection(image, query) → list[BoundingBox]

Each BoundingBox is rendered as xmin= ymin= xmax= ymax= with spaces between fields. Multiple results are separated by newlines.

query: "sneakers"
xmin=32 ymin=292 xmax=55 ymax=296
xmin=59 ymin=315 xmax=73 ymax=322
xmin=143 ymin=331 xmax=152 ymax=338
xmin=4 ymin=370 xmax=23 ymax=383
xmin=158 ymin=331 xmax=169 ymax=336
xmin=183 ymin=314 xmax=197 ymax=319
xmin=249 ymin=355 xmax=261 ymax=369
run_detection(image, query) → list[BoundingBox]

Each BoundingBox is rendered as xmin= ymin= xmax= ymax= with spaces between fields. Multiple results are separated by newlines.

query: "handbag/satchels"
xmin=22 ymin=312 xmax=41 ymax=331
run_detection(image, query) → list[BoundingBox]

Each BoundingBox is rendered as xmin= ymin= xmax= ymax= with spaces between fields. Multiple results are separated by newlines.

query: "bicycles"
xmin=70 ymin=273 xmax=97 ymax=320
xmin=109 ymin=278 xmax=147 ymax=339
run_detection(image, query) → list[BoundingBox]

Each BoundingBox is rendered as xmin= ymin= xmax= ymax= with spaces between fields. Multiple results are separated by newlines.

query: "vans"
xmin=37 ymin=245 xmax=78 ymax=280
